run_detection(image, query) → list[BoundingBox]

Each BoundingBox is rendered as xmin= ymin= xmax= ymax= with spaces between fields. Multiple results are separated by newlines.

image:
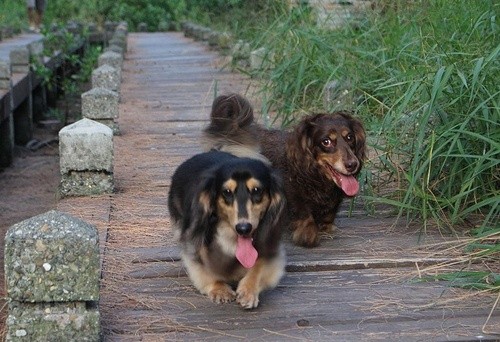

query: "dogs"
xmin=168 ymin=149 xmax=286 ymax=308
xmin=198 ymin=94 xmax=365 ymax=247
